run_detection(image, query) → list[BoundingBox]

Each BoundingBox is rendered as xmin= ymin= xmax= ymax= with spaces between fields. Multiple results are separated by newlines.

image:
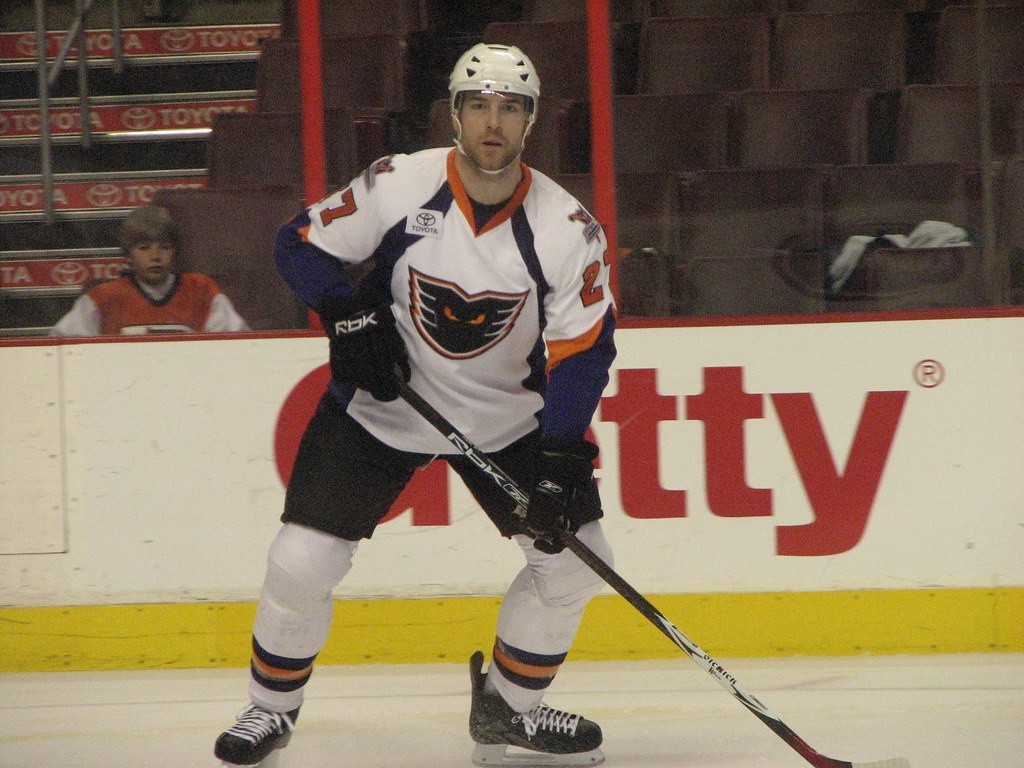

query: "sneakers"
xmin=213 ymin=698 xmax=299 ymax=768
xmin=469 ymin=651 xmax=605 ymax=766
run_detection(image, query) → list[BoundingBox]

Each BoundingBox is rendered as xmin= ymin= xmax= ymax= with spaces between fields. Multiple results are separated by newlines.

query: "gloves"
xmin=319 ymin=290 xmax=411 ymax=401
xmin=510 ymin=437 xmax=603 ymax=555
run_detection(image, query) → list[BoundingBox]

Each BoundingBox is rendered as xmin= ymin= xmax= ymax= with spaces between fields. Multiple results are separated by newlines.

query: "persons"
xmin=214 ymin=44 xmax=617 ymax=765
xmin=48 ymin=204 xmax=253 ymax=336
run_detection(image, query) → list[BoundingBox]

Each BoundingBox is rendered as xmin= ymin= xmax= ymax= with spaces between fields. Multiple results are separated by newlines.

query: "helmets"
xmin=449 ymin=43 xmax=540 ymax=123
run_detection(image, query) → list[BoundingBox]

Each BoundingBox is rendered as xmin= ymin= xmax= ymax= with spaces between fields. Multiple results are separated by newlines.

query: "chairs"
xmin=152 ymin=0 xmax=1024 ymax=332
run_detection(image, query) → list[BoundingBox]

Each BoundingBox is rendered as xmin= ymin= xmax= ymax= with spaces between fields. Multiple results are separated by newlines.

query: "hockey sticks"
xmin=396 ymin=377 xmax=911 ymax=768
